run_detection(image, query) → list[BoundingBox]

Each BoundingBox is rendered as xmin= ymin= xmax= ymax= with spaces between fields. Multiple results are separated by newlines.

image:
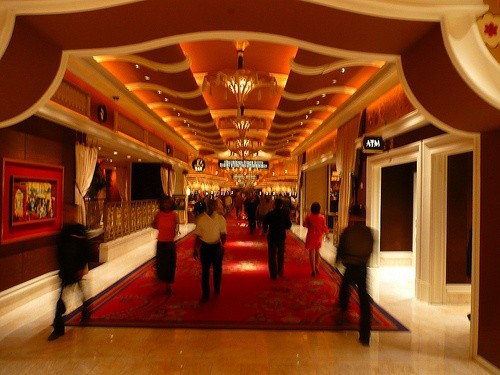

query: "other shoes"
xmin=270 ymin=272 xmax=285 ymax=281
xmin=359 ymin=337 xmax=370 ymax=346
xmin=201 ymin=294 xmax=210 ymax=303
xmin=81 ymin=312 xmax=89 ymax=321
xmin=166 ymin=287 xmax=171 ymax=295
xmin=49 ymin=330 xmax=65 ymax=340
xmin=312 ymin=268 xmax=319 ymax=277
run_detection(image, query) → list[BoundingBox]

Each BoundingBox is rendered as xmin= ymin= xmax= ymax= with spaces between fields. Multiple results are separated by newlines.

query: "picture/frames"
xmin=11 ymin=175 xmax=57 ymax=226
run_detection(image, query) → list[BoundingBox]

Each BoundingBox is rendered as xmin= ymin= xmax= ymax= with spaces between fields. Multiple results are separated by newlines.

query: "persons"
xmin=47 ymin=204 xmax=92 ymax=340
xmin=334 ymin=205 xmax=373 ymax=345
xmin=193 ymin=200 xmax=227 ymax=304
xmin=188 ymin=187 xmax=299 ymax=234
xmin=303 ymin=202 xmax=327 ymax=277
xmin=262 ymin=198 xmax=292 ymax=280
xmin=150 ymin=196 xmax=180 ymax=296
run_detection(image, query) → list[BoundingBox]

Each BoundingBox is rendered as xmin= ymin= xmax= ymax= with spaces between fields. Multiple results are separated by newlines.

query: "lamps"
xmin=202 ymin=39 xmax=290 ymax=187
